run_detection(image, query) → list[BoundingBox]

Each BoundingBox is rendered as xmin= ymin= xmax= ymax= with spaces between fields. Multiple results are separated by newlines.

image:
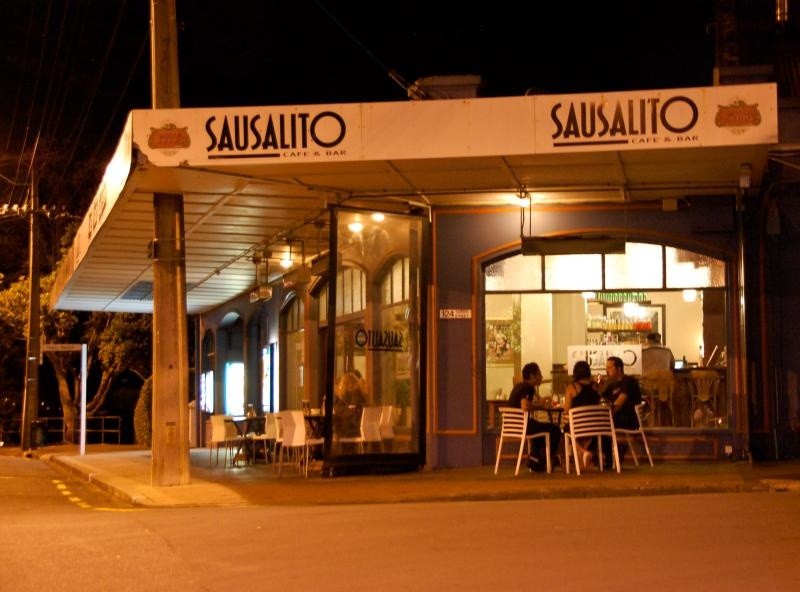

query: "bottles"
xmin=590 ymin=316 xmax=651 ymax=330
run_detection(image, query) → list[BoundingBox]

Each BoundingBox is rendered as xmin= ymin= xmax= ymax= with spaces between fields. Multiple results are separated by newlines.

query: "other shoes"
xmin=528 ymin=458 xmax=545 ymax=472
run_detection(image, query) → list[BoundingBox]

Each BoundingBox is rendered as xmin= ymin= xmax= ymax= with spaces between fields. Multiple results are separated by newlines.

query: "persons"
xmin=334 ymin=370 xmax=372 ymax=438
xmin=507 ymin=362 xmax=562 ymax=473
xmin=562 ymin=356 xmax=642 ymax=473
xmin=640 ymin=332 xmax=676 ymax=419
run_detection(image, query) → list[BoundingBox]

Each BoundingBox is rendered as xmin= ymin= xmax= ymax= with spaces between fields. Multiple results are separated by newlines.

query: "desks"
xmin=304 ymin=415 xmax=324 ymax=465
xmin=224 ymin=415 xmax=264 ymax=464
xmin=536 ymin=404 xmax=566 ymax=461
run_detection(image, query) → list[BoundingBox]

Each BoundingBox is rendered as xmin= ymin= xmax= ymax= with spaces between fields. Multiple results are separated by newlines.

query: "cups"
xmin=551 ymin=394 xmax=565 ymax=407
xmin=595 ymin=374 xmax=604 ymax=384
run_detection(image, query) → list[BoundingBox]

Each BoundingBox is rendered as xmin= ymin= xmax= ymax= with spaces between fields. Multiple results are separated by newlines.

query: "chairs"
xmin=564 ymin=404 xmax=621 ymax=475
xmin=639 ymin=370 xmax=674 ymax=428
xmin=613 ymin=401 xmax=654 ymax=469
xmin=494 ymin=406 xmax=552 ymax=476
xmin=207 ymin=406 xmax=398 ymax=478
xmin=687 ymin=369 xmax=720 ymax=427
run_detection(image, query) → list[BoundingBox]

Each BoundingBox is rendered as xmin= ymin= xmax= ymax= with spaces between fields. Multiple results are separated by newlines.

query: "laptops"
xmin=674 ymin=360 xmax=685 ymax=373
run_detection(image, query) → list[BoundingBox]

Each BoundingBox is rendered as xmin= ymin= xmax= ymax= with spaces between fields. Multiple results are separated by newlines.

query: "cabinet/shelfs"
xmin=586 ymin=300 xmax=665 ymax=350
